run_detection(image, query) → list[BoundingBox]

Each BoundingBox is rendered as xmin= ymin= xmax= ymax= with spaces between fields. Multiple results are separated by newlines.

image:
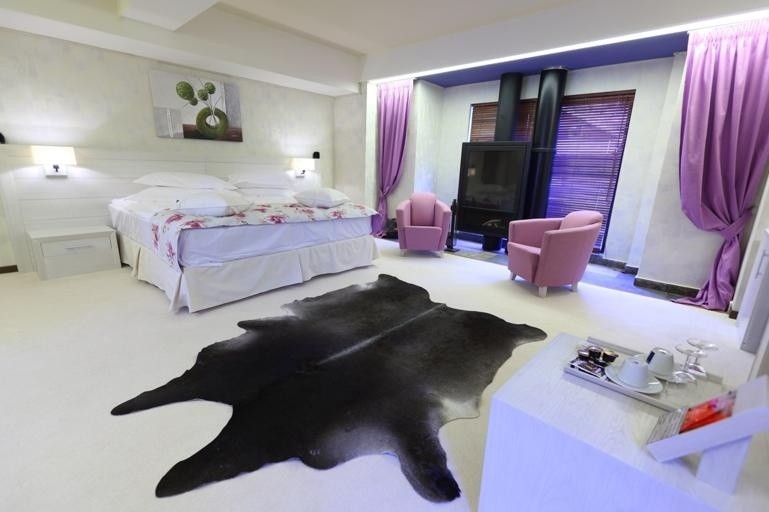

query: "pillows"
xmin=503 ymin=207 xmax=604 ymax=299
xmin=134 ymin=171 xmax=237 ymax=194
xmin=292 ymin=186 xmax=350 ymax=209
xmin=128 ymin=189 xmax=183 ymax=216
xmin=170 ymin=191 xmax=254 ymax=217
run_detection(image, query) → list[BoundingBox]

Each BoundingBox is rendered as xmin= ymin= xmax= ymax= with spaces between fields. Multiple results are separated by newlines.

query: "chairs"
xmin=395 ymin=189 xmax=454 ymax=253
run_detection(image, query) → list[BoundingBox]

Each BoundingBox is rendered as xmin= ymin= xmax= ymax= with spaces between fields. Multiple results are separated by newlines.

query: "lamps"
xmin=311 ymin=151 xmax=322 ymax=170
xmin=29 ymin=143 xmax=79 ymax=181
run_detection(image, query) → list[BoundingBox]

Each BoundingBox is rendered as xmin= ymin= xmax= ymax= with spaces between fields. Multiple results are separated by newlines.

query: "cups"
xmin=618 ymin=357 xmax=650 ymax=389
xmin=646 ymin=346 xmax=674 ymax=376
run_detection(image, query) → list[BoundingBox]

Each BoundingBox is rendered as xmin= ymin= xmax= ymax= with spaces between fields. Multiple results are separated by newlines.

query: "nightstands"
xmin=24 ymin=224 xmax=124 ymax=283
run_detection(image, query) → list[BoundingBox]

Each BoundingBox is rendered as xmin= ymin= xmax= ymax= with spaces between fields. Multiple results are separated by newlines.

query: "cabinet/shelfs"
xmin=454 ymin=139 xmax=533 ymax=240
xmin=475 ymin=331 xmax=767 ymax=510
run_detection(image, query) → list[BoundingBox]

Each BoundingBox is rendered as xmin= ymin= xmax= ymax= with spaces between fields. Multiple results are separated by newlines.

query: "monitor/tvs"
xmin=456 ymin=145 xmax=526 ymax=215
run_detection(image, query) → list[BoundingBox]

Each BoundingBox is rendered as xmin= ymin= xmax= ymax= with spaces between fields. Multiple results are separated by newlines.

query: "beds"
xmin=106 ymin=186 xmax=382 ymax=316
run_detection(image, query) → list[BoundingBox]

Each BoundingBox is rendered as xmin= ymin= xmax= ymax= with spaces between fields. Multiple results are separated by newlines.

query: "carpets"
xmin=108 ymin=271 xmax=551 ymax=507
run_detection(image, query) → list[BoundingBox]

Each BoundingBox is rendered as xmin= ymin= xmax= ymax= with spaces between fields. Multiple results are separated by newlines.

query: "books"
xmin=645 ymin=372 xmax=769 ymax=464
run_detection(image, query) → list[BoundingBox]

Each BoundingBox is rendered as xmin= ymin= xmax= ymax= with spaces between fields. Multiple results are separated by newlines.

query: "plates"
xmin=605 ymin=362 xmax=663 ymax=396
xmin=634 ymin=353 xmax=687 ymax=381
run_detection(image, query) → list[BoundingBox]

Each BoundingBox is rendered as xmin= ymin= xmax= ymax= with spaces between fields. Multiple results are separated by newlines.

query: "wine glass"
xmin=684 ymin=337 xmax=718 ymax=393
xmin=665 ymin=344 xmax=708 ymax=407
